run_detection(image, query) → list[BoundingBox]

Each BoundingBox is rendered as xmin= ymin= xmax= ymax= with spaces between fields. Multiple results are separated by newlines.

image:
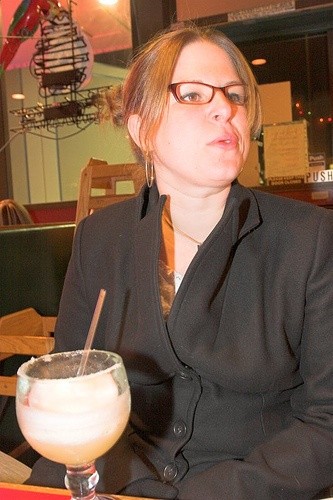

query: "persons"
xmin=20 ymin=25 xmax=333 ymax=500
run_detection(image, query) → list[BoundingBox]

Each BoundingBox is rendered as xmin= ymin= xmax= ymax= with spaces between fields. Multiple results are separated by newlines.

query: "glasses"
xmin=168 ymin=82 xmax=250 ymax=107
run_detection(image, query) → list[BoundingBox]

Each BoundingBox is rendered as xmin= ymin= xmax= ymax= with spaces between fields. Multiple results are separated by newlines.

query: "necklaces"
xmin=162 ymin=214 xmax=202 ymax=250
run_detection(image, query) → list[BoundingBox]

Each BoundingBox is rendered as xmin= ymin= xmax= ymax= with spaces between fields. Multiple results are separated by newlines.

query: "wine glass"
xmin=16 ymin=350 xmax=131 ymax=499
